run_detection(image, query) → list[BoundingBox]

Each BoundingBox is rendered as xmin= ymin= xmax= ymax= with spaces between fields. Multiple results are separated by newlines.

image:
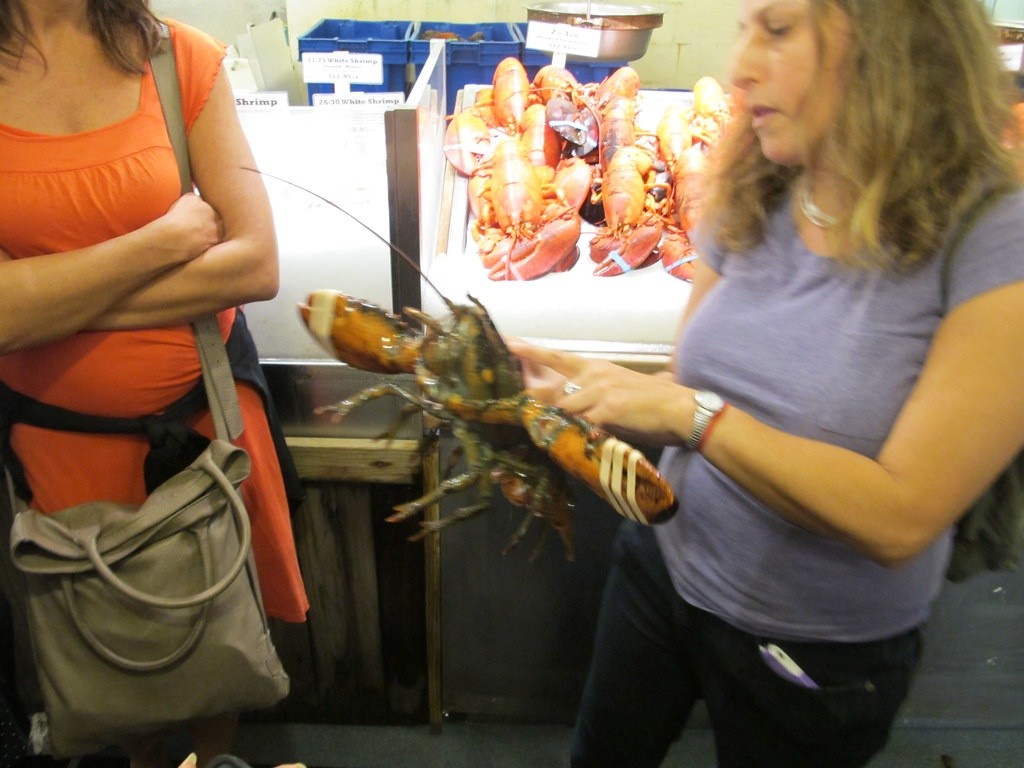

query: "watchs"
xmin=685 ymin=384 xmax=726 ymax=450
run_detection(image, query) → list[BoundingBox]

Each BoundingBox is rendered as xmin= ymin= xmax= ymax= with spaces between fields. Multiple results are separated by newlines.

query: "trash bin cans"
xmin=297 ymin=18 xmax=628 ymax=108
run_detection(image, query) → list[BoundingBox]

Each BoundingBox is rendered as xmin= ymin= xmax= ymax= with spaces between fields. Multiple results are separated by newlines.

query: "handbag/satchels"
xmin=9 ymin=440 xmax=290 ymax=760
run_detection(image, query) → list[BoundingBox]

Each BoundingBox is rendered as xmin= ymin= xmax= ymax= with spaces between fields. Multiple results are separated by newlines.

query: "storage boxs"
xmin=296 ymin=18 xmax=627 ymax=105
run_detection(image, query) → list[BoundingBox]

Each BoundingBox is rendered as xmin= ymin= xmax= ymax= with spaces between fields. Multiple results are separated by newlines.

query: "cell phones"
xmin=759 ymin=643 xmax=820 ymax=690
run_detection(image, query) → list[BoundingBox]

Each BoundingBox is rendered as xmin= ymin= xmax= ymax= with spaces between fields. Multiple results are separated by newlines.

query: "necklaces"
xmin=799 ymin=170 xmax=853 ymax=230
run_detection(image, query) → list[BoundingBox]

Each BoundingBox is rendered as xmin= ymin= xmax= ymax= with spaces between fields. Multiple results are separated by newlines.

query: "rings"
xmin=564 ymin=379 xmax=581 ymax=396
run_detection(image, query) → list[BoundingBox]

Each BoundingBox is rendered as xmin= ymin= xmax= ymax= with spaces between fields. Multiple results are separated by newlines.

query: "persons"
xmin=1 ymin=0 xmax=312 ymax=767
xmin=481 ymin=0 xmax=1024 ymax=768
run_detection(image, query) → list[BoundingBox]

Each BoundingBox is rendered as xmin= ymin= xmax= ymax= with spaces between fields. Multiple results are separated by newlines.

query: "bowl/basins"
xmin=523 ymin=3 xmax=663 ymax=63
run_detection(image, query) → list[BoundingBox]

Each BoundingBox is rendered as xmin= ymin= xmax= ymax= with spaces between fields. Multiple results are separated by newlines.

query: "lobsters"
xmin=235 ymin=165 xmax=679 ymax=569
xmin=441 ymin=56 xmax=728 ymax=284
